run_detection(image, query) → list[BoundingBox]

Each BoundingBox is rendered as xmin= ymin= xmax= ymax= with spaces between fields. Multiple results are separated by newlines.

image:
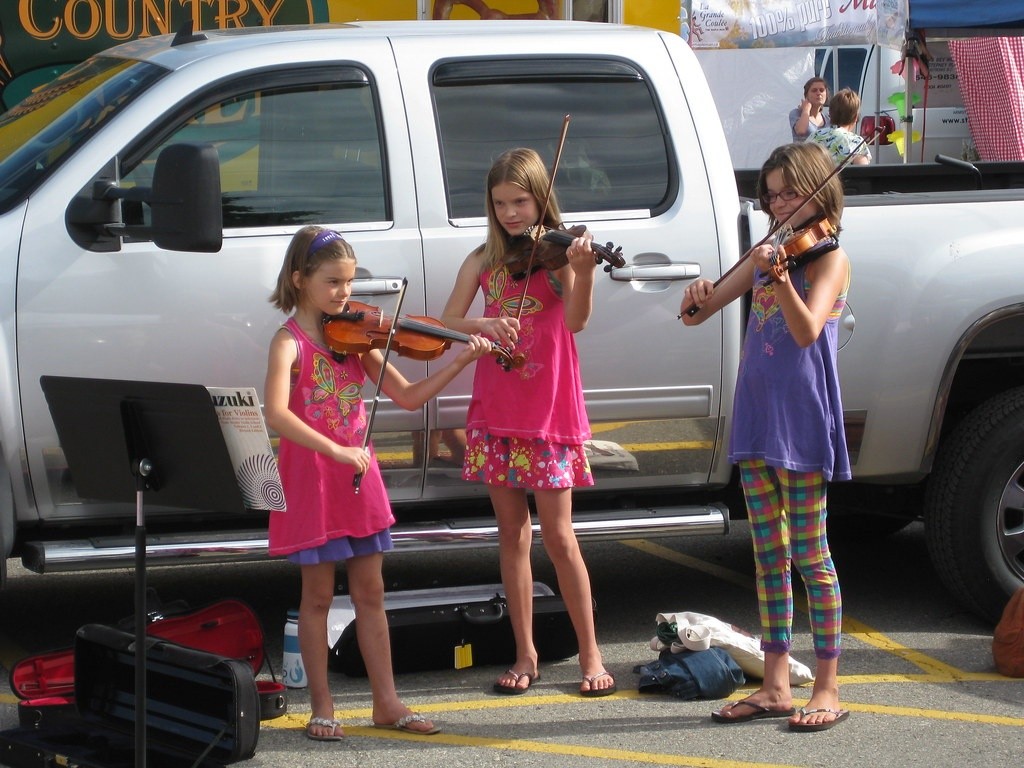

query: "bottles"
xmin=282 ymin=608 xmax=308 ymax=688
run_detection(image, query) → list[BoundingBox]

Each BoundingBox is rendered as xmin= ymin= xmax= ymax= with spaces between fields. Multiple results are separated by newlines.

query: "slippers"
xmin=712 ymin=699 xmax=795 ymax=722
xmin=790 ymin=706 xmax=849 ymax=729
xmin=307 ymin=717 xmax=343 ymax=741
xmin=374 ymin=711 xmax=442 ymax=734
xmin=580 ymin=671 xmax=616 ymax=696
xmin=496 ymin=668 xmax=540 ymax=694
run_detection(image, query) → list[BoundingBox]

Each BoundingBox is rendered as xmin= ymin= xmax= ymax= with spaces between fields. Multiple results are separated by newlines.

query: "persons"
xmin=805 ymin=85 xmax=872 ymax=167
xmin=264 ymin=224 xmax=492 ymax=741
xmin=681 ymin=140 xmax=852 ymax=732
xmin=789 ymin=78 xmax=830 ymax=142
xmin=438 ymin=147 xmax=617 ymax=696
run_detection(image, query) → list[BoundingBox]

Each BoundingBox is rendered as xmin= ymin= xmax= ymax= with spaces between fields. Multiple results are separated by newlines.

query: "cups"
xmin=255 ymin=677 xmax=288 ymax=721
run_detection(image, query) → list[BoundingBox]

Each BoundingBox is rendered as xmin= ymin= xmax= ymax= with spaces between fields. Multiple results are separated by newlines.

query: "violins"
xmin=504 ymin=223 xmax=626 ymax=273
xmin=758 ymin=213 xmax=836 ymax=287
xmin=323 ymin=300 xmax=526 ymax=371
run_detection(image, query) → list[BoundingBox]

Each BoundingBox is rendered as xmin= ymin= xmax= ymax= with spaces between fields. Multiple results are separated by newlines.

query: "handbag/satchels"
xmin=633 ymin=645 xmax=745 ymax=698
xmin=651 ymin=611 xmax=815 ymax=686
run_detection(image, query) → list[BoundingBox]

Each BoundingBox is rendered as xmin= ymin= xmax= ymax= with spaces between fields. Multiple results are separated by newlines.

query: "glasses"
xmin=762 ymin=187 xmax=799 ymax=204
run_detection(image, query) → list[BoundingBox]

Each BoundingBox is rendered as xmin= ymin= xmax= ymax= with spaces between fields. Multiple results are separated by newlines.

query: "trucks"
xmin=683 ymin=42 xmax=916 ymax=166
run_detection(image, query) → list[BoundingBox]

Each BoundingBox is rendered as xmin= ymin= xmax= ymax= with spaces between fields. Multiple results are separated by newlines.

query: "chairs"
xmin=333 ymin=108 xmax=383 ymax=220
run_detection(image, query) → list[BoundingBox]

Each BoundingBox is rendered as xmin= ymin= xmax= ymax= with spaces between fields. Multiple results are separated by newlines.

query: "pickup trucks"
xmin=1 ymin=18 xmax=1022 ymax=632
